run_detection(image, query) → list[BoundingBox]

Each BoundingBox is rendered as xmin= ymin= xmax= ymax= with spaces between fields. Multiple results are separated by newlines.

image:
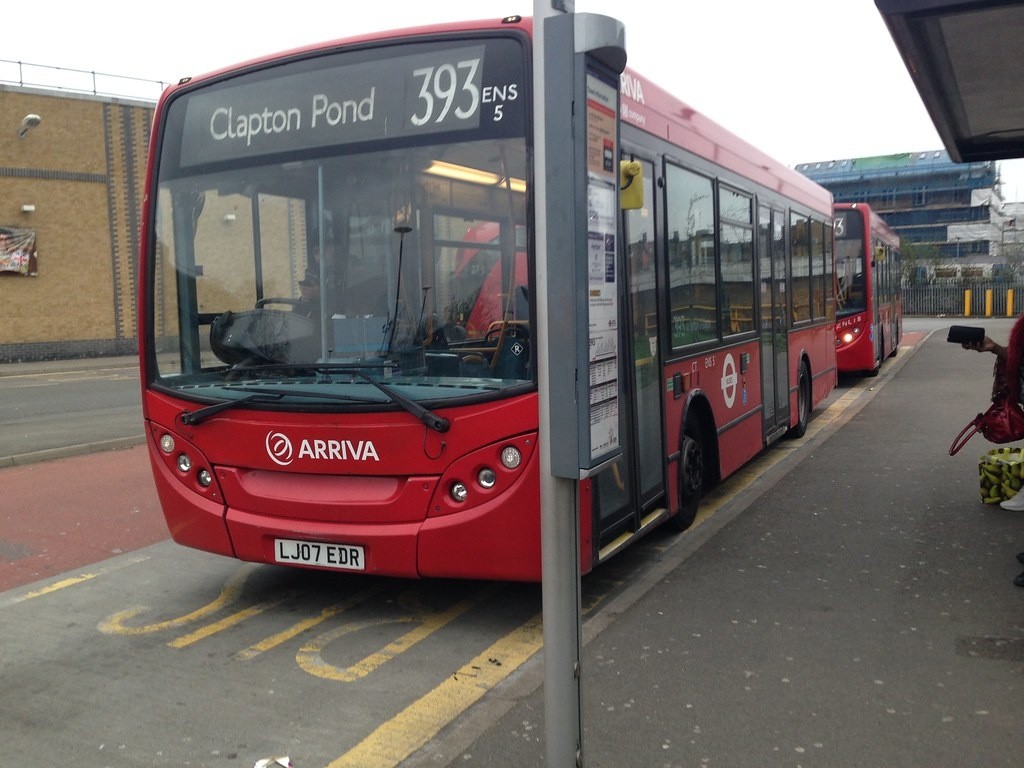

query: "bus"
xmin=134 ymin=9 xmax=841 ymax=586
xmin=834 ymin=202 xmax=904 ymax=378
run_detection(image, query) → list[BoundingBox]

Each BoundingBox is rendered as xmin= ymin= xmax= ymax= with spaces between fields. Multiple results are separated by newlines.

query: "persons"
xmin=292 ymin=224 xmax=383 ymax=316
xmin=963 ymin=311 xmax=1024 ymax=586
